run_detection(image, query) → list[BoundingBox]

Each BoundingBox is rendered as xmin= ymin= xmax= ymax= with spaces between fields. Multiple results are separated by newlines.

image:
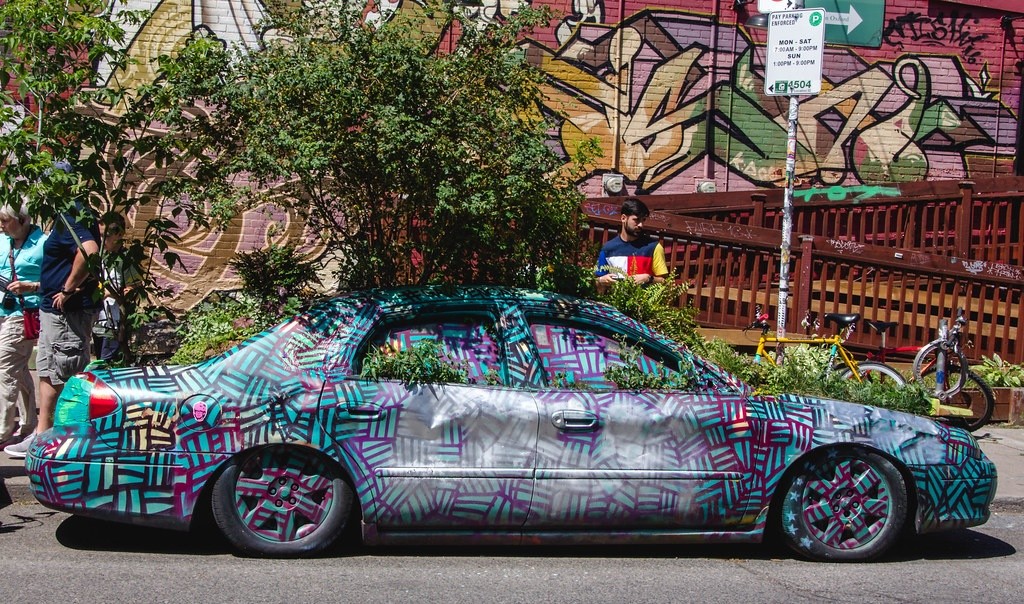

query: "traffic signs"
xmin=802 ymin=0 xmax=887 ymax=49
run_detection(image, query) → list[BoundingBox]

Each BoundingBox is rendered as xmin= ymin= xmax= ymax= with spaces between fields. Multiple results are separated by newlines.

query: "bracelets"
xmin=35 ymin=285 xmax=38 ymax=291
xmin=648 ymin=276 xmax=654 ymax=283
xmin=61 ymin=286 xmax=76 ymax=295
xmin=599 ymin=277 xmax=603 ymax=286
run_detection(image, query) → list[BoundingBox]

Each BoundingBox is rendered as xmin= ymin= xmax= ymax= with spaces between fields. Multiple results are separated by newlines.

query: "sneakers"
xmin=4 ymin=429 xmax=56 ymax=456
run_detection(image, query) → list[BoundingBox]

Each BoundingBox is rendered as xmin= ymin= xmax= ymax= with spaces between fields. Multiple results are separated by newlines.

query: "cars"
xmin=25 ymin=283 xmax=999 ymax=561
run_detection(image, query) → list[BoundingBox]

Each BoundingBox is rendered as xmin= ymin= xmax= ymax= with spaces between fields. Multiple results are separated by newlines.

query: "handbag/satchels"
xmin=22 ymin=308 xmax=41 ymax=340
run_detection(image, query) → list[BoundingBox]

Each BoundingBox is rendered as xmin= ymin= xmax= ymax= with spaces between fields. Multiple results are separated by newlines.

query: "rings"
xmin=14 ymin=292 xmax=17 ymax=293
xmin=606 ymin=280 xmax=608 ymax=283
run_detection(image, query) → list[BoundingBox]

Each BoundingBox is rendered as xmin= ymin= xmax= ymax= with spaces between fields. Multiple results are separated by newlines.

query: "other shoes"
xmin=0 ymin=439 xmax=17 ymax=450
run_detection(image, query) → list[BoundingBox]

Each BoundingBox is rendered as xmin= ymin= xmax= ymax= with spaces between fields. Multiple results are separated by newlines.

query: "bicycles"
xmin=849 ymin=305 xmax=997 ymax=433
xmin=740 ymin=303 xmax=908 ymax=393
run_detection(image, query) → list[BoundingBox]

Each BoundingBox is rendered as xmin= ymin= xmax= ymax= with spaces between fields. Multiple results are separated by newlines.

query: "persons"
xmin=0 ymin=195 xmax=49 ymax=452
xmin=91 ymin=212 xmax=143 ymax=362
xmin=4 ymin=162 xmax=100 ymax=457
xmin=595 ymin=198 xmax=669 ymax=296
